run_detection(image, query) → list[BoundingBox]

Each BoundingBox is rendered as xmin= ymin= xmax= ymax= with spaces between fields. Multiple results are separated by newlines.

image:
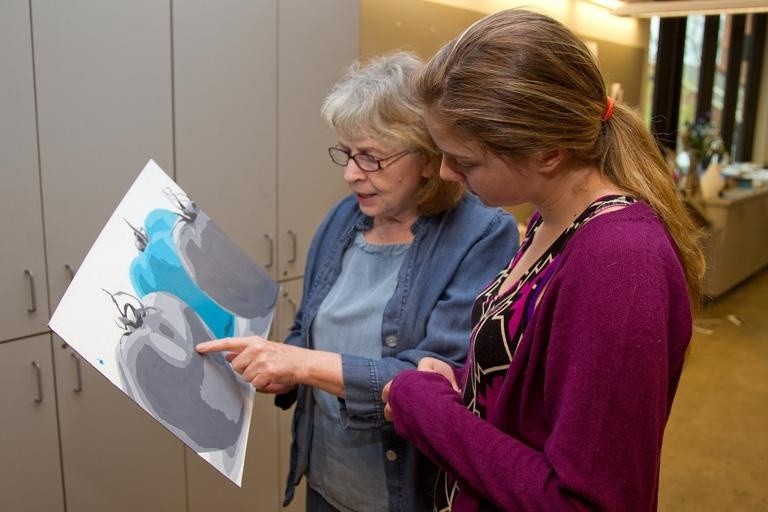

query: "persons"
xmin=379 ymin=4 xmax=715 ymax=510
xmin=193 ymin=49 xmax=522 ymax=511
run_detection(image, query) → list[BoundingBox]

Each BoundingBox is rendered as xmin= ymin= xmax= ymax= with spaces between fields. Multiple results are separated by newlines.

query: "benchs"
xmin=689 ymin=185 xmax=768 ymax=299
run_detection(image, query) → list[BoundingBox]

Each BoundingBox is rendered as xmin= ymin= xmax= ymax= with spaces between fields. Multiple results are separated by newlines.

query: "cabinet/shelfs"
xmin=3 ymin=4 xmax=175 ymax=343
xmin=162 ymin=277 xmax=317 ymax=510
xmin=1 ymin=331 xmax=192 ymax=511
xmin=167 ymin=2 xmax=358 ymax=282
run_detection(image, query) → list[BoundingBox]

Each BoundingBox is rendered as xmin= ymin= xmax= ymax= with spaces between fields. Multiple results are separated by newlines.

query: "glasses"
xmin=328 ymin=143 xmax=420 ymax=173
